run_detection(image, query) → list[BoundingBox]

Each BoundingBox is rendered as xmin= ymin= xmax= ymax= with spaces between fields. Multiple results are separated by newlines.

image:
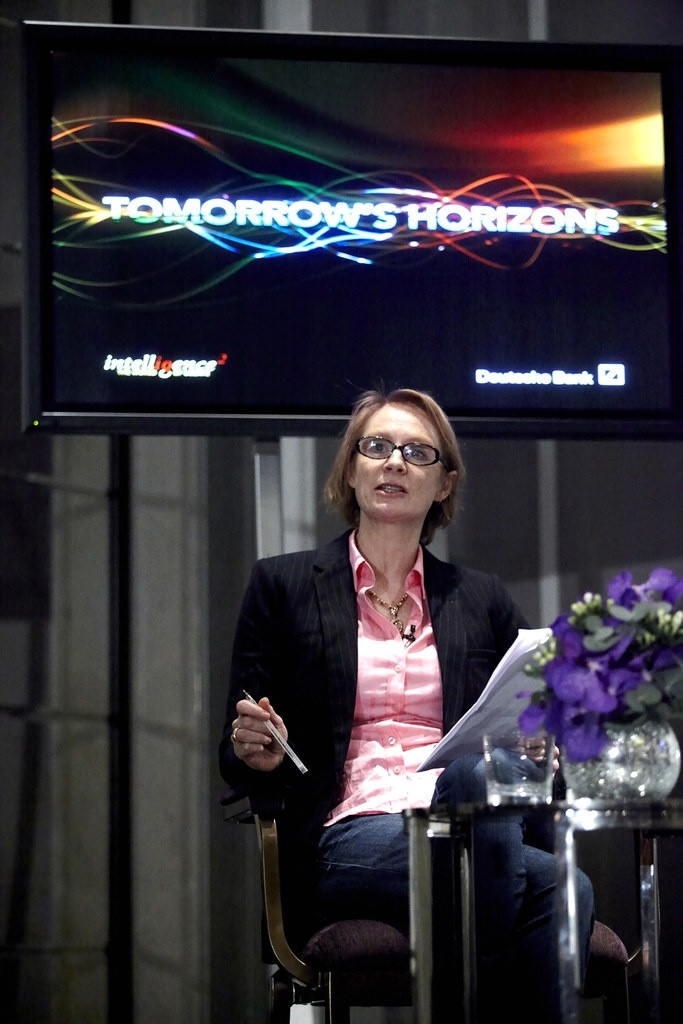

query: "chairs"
xmin=220 ymin=789 xmax=630 ymax=1024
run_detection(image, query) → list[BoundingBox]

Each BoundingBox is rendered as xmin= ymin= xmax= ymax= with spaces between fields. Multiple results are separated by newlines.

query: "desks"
xmin=403 ymin=795 xmax=683 ymax=1024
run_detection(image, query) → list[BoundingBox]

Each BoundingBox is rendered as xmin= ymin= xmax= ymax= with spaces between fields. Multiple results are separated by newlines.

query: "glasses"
xmin=353 ymin=435 xmax=451 ymax=470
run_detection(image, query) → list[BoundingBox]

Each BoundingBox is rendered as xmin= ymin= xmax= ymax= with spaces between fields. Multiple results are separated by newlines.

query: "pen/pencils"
xmin=242 ymin=689 xmax=309 ymax=774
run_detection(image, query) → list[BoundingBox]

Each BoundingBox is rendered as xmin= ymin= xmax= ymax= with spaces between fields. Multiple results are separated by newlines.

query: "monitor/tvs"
xmin=21 ymin=20 xmax=682 ymax=441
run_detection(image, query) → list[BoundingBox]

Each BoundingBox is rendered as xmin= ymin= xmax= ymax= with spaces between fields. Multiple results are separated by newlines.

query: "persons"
xmin=220 ymin=388 xmax=594 ymax=1024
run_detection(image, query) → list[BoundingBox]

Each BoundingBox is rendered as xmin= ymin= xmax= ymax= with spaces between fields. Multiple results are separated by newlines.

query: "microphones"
xmin=411 ymin=625 xmax=416 ymax=633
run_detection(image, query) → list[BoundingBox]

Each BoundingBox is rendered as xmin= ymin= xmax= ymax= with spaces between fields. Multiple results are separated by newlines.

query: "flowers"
xmin=514 ymin=567 xmax=683 ymax=763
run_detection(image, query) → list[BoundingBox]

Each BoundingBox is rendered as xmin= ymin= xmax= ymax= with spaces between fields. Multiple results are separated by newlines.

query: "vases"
xmin=561 ymin=718 xmax=680 ymax=803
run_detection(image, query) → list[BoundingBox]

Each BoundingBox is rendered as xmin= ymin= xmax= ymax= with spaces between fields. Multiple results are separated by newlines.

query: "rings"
xmin=233 ymin=728 xmax=244 ymax=745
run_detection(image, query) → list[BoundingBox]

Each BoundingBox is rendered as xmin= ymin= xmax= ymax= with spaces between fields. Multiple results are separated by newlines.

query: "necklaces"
xmin=368 ymin=590 xmax=409 ymax=631
